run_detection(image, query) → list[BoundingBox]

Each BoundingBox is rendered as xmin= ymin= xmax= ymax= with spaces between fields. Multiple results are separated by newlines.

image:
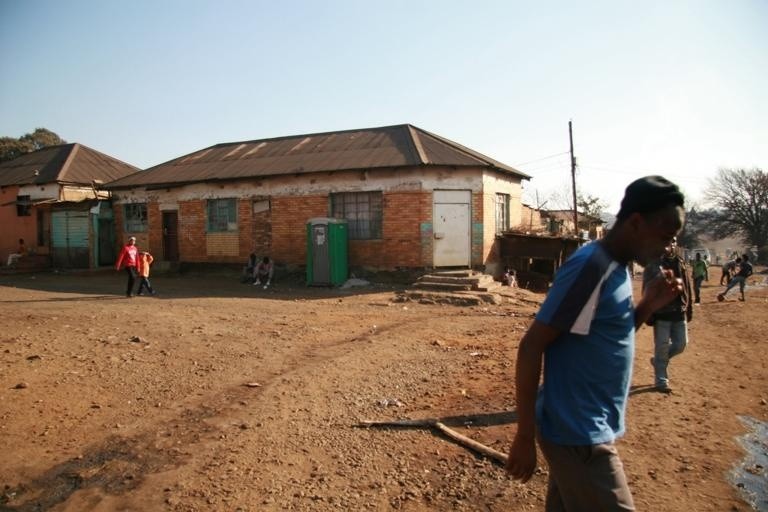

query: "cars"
xmin=689 ymin=247 xmax=711 ymax=265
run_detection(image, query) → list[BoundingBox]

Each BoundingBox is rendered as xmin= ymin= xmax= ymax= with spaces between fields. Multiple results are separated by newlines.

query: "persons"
xmin=720 ymin=257 xmax=741 ymax=285
xmin=252 ymin=255 xmax=275 ymax=289
xmin=508 ymin=269 xmax=518 ymax=287
xmin=115 ymin=235 xmax=140 ymax=297
xmin=703 ymin=254 xmax=711 ymax=280
xmin=719 ymin=253 xmax=754 ymax=301
xmin=691 ymin=252 xmax=709 ymax=306
xmin=7 ymin=238 xmax=28 ymax=265
xmin=505 ymin=173 xmax=688 ymax=511
xmin=137 ymin=251 xmax=159 ymax=296
xmin=500 ymin=266 xmax=509 ymax=285
xmin=240 ymin=253 xmax=260 ymax=284
xmin=640 ymin=236 xmax=693 ymax=392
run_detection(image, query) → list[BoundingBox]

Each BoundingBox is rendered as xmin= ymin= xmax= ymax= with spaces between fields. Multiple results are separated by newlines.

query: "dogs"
xmin=504 ymin=267 xmax=520 ymax=287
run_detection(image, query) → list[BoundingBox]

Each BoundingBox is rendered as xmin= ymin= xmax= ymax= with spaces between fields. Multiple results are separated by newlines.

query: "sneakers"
xmin=655 ymin=383 xmax=671 ymax=394
